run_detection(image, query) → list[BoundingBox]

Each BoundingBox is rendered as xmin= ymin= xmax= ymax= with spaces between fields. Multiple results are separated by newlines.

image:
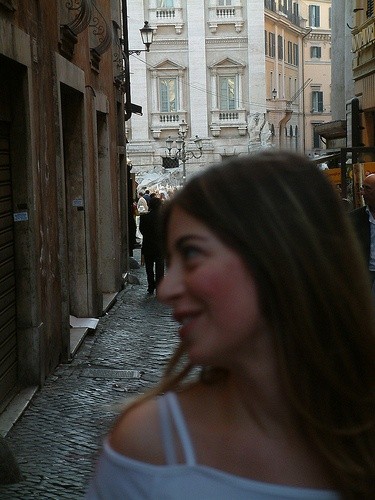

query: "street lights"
xmin=166 ymin=119 xmax=204 ymax=188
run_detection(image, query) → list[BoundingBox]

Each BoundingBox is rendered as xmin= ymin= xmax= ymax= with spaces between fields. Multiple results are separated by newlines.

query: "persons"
xmin=85 ymin=148 xmax=375 ymax=500
xmin=139 ymin=197 xmax=165 ymax=294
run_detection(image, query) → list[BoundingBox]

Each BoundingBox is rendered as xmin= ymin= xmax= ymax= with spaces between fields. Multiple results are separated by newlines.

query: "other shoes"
xmin=147 ymin=288 xmax=154 ymax=294
